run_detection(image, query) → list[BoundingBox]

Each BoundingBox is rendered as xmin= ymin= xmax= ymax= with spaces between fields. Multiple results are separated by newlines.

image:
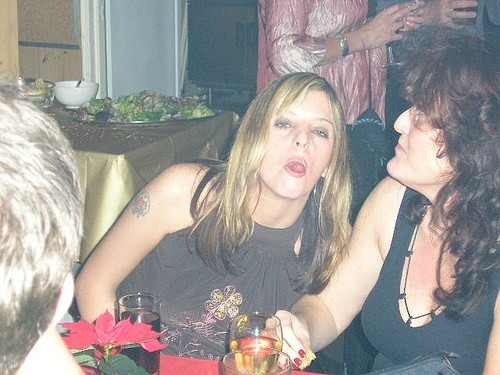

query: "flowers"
xmin=56 ymin=307 xmax=171 ymax=375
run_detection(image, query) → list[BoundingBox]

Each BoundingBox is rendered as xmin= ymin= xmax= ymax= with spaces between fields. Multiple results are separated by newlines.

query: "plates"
xmin=67 ymin=108 xmax=218 ymax=126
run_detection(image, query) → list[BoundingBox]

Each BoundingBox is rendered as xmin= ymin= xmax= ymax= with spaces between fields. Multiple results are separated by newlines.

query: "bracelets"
xmin=336 ymin=31 xmax=350 ymax=58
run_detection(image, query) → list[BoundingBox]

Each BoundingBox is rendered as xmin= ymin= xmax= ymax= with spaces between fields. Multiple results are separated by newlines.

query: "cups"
xmin=118 ymin=293 xmax=161 ymax=375
xmin=222 ymin=348 xmax=292 ymax=375
xmin=229 ymin=311 xmax=283 ymax=375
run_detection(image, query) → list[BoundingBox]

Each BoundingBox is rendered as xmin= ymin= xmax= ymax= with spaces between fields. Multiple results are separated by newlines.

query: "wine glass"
xmin=376 ymin=5 xmax=405 ymax=69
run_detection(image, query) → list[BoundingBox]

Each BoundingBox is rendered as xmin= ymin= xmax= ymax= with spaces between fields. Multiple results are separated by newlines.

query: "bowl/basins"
xmin=0 ymin=78 xmax=55 ymax=111
xmin=54 ymin=81 xmax=99 ymax=109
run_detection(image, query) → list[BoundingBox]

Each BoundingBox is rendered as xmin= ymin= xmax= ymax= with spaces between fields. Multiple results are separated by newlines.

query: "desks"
xmin=156 ymin=352 xmax=323 ymax=375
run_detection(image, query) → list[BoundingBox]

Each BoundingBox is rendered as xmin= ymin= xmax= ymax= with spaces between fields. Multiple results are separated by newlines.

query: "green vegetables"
xmin=76 ymin=91 xmax=208 ymax=122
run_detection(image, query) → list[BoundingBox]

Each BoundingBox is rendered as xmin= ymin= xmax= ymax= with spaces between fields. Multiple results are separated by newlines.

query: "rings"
xmin=392 ymin=24 xmax=398 ymax=31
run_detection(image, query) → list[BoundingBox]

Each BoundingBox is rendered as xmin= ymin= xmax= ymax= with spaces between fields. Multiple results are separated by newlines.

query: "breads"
xmin=299 ymin=349 xmax=316 ymax=371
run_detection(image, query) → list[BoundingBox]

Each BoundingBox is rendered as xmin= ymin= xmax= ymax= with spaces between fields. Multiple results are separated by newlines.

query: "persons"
xmin=257 ymin=0 xmax=500 ymax=125
xmin=262 ymin=24 xmax=500 ymax=375
xmin=0 ymin=93 xmax=87 ymax=375
xmin=73 ymin=72 xmax=353 ymax=375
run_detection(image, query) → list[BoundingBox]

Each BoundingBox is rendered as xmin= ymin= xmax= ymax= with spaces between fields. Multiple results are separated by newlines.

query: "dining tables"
xmin=41 ymin=104 xmax=242 ymax=262
